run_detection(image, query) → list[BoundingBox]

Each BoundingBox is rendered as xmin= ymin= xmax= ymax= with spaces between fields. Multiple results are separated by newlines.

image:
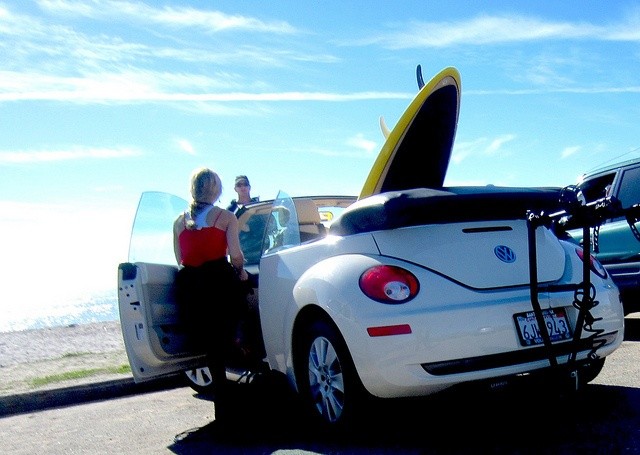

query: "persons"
xmin=174 ymin=168 xmax=254 ymax=430
xmin=226 ymin=177 xmax=259 ymax=218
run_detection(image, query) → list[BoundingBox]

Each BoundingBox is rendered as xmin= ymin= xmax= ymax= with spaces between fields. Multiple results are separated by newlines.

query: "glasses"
xmin=236 ymin=182 xmax=249 ymax=187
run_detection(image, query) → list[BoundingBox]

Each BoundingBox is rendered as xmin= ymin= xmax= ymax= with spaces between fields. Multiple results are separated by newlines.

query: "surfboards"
xmin=359 ymin=65 xmax=461 ymax=203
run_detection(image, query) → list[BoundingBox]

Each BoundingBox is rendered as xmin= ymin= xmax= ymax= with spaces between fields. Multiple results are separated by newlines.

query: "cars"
xmin=118 ymin=188 xmax=625 ymax=447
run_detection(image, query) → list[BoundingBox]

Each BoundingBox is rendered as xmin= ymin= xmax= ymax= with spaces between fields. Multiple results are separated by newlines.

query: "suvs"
xmin=549 ymin=148 xmax=640 ymax=316
